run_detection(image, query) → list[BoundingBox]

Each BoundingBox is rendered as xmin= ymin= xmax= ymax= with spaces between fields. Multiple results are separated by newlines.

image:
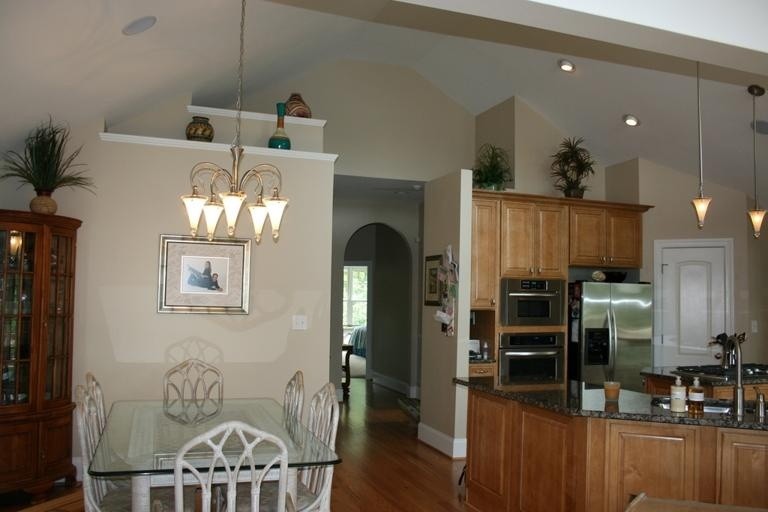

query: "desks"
xmin=342 ymin=343 xmax=353 ymax=400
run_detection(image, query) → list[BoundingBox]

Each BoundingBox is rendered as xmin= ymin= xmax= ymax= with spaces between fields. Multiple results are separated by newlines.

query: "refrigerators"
xmin=570 ymin=280 xmax=653 ymax=393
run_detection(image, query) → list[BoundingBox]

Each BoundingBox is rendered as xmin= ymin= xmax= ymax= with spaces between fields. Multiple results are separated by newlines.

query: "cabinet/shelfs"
xmin=501 ymin=194 xmax=654 ymax=278
xmin=96 ymin=92 xmax=337 ymax=164
xmin=0 ymin=210 xmax=82 ymax=505
xmin=468 ymin=188 xmax=500 ymax=391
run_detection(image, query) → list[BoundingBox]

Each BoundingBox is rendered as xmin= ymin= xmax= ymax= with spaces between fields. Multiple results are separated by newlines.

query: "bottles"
xmin=669 ymin=373 xmax=687 ymax=413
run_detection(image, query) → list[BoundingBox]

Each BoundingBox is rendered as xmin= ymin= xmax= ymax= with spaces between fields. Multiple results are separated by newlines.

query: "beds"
xmin=348 ymin=324 xmax=367 ymax=358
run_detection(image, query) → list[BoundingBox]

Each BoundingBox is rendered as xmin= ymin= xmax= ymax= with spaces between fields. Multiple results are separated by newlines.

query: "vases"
xmin=186 ymin=116 xmax=214 ymax=141
xmin=269 ymin=93 xmax=311 ymax=151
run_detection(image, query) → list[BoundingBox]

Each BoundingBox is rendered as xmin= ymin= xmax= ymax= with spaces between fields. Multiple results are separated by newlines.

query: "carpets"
xmin=396 ymin=396 xmax=420 ymax=423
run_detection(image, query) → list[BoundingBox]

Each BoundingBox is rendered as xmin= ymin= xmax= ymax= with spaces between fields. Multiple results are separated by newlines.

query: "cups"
xmin=605 ymin=401 xmax=619 ymax=413
xmin=603 ymin=381 xmax=621 ymax=401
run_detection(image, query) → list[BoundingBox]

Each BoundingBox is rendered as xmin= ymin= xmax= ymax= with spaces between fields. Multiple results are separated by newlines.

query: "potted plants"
xmin=547 ymin=136 xmax=596 ymax=198
xmin=1 ymin=113 xmax=96 ymax=215
xmin=470 ymin=143 xmax=513 ymax=191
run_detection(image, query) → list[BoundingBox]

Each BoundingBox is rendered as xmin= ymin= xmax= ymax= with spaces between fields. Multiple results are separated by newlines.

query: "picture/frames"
xmin=156 ymin=234 xmax=252 ymax=316
xmin=423 ymin=255 xmax=443 ymax=307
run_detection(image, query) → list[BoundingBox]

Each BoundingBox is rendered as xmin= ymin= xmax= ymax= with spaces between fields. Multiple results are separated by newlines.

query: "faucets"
xmin=720 ymin=339 xmax=747 ymax=418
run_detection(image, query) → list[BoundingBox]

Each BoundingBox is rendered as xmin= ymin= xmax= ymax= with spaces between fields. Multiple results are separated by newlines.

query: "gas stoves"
xmin=676 ymin=363 xmax=768 ymax=383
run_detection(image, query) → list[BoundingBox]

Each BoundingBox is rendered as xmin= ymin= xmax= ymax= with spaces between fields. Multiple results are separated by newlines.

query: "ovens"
xmin=499 ymin=331 xmax=566 ymax=384
xmin=500 ymin=279 xmax=566 ymax=326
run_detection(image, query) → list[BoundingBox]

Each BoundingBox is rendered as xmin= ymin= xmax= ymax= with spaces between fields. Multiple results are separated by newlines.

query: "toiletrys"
xmin=671 ymin=375 xmax=686 ymax=412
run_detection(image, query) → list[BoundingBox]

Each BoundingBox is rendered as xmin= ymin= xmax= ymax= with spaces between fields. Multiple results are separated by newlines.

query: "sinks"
xmin=655 ymin=395 xmax=768 ymax=414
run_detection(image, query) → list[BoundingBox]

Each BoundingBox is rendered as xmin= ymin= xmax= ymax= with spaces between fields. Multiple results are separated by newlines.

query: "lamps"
xmin=690 ymin=61 xmax=713 ymax=230
xmin=181 ymin=1 xmax=291 ymax=242
xmin=624 ymin=114 xmax=639 ymax=127
xmin=560 ymin=58 xmax=575 ymax=73
xmin=745 ymin=85 xmax=767 ymax=238
